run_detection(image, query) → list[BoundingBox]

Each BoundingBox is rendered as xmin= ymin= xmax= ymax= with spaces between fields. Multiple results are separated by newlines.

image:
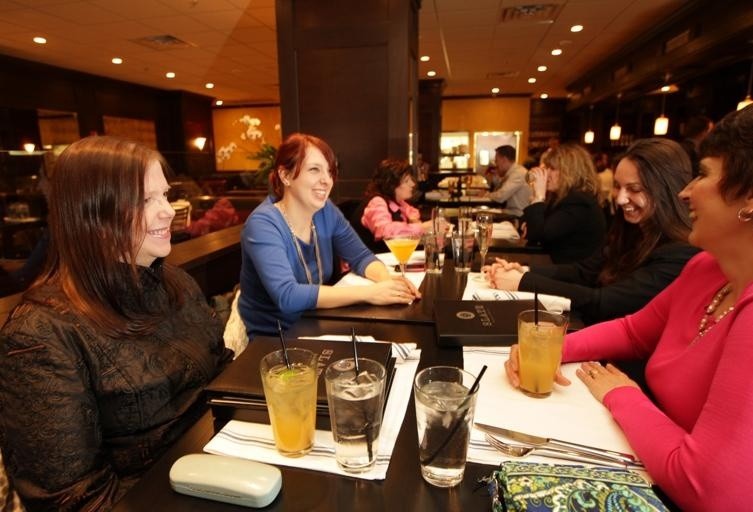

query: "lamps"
xmin=653 ymin=95 xmax=670 ymax=137
xmin=584 ymin=109 xmax=594 ymax=145
xmin=608 ymin=99 xmax=623 ymax=143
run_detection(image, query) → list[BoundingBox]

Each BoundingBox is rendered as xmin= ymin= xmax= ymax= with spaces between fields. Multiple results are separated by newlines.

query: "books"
xmin=433 ymin=299 xmax=549 ymax=347
xmin=205 ymin=335 xmax=397 ymax=432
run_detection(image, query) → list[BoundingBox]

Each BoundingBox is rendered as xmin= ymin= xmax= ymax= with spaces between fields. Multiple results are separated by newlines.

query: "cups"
xmin=451 ymin=232 xmax=475 ymax=272
xmin=324 ymin=358 xmax=388 ymax=473
xmin=517 ymin=309 xmax=569 ymax=399
xmin=259 ymin=347 xmax=318 ymax=458
xmin=414 ymin=366 xmax=479 ymax=489
xmin=423 ymin=232 xmax=446 ymax=275
xmin=447 ymin=180 xmax=454 ymax=198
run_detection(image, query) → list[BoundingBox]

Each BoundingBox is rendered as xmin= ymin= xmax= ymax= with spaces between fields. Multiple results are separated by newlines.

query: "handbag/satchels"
xmin=487 ymin=459 xmax=672 ymax=512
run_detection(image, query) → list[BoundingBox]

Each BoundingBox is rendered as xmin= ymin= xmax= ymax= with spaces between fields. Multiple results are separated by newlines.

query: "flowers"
xmin=247 ymin=137 xmax=279 ymax=184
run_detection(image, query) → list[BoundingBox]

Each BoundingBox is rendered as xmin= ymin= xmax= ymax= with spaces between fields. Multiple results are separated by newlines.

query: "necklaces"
xmin=280 ymin=202 xmax=323 ymax=287
xmin=698 ymin=279 xmax=743 ymax=339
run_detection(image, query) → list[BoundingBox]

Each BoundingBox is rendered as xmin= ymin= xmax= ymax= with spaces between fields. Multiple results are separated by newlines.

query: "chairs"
xmin=170 ymin=207 xmax=188 ymax=242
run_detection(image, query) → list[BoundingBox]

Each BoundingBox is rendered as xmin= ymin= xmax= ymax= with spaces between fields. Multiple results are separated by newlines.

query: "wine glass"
xmin=475 ymin=212 xmax=493 ymax=283
xmin=457 ymin=206 xmax=472 ymax=235
xmin=383 ymin=234 xmax=422 ymax=277
xmin=431 ymin=207 xmax=447 ymax=269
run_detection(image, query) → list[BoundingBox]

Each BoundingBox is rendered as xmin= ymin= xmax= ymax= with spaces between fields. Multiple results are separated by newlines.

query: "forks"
xmin=484 ymin=432 xmax=626 ymax=465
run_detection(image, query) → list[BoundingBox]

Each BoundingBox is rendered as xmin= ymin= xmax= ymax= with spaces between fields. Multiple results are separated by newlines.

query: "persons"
xmin=0 ymin=134 xmax=236 ymax=512
xmin=481 ymin=138 xmax=702 ymax=318
xmin=479 ymin=91 xmax=753 ymax=246
xmin=221 ymin=133 xmax=422 ymax=339
xmin=507 ymin=102 xmax=753 ymax=512
xmin=339 ymin=158 xmax=450 ymax=276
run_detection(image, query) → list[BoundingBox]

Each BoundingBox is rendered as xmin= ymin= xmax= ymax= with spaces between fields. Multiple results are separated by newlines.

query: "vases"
xmin=268 ymin=170 xmax=278 ymax=196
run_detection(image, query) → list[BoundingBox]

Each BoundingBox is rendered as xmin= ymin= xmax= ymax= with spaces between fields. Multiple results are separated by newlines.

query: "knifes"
xmin=473 ymin=422 xmax=635 ymax=465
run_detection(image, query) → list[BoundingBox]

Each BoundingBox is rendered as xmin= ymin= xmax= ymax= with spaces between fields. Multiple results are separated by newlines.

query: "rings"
xmin=397 ymin=292 xmax=400 ymax=296
xmin=588 ymin=367 xmax=598 ymax=375
